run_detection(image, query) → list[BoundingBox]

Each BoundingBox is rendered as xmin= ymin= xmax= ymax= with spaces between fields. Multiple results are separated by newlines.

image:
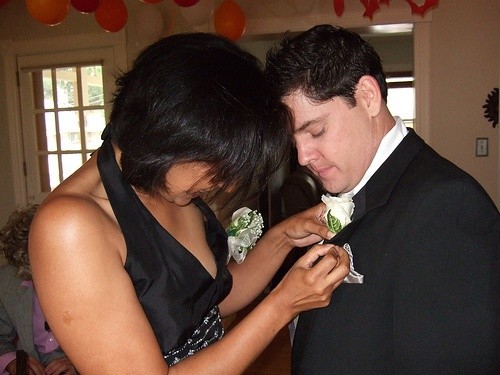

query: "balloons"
xmin=26 ymin=0 xmax=246 ymax=40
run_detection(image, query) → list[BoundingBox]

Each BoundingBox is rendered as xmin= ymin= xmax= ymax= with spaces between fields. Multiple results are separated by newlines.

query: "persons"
xmin=1 ymin=203 xmax=78 ymax=375
xmin=27 ymin=30 xmax=350 ymax=374
xmin=266 ymin=23 xmax=500 ymax=374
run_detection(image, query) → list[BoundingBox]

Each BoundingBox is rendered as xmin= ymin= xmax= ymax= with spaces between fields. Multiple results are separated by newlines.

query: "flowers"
xmin=225 ymin=207 xmax=264 ymax=252
xmin=321 ymin=193 xmax=355 ymax=233
